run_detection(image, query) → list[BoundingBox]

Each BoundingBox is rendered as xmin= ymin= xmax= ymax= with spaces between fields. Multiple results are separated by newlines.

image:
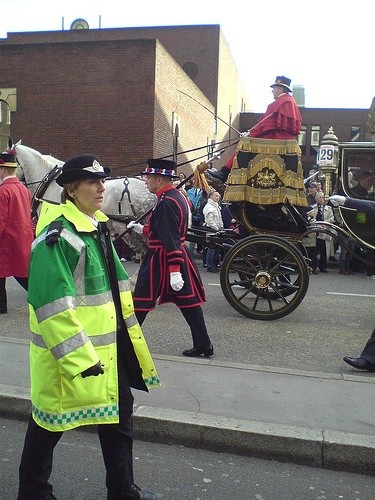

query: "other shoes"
xmin=320 ymin=268 xmax=328 ymax=273
xmin=120 ymin=257 xmax=127 ymax=262
xmin=207 ymin=269 xmax=220 ymax=273
xmin=339 ymin=269 xmax=356 ymax=275
xmin=329 ymin=256 xmax=338 ymax=262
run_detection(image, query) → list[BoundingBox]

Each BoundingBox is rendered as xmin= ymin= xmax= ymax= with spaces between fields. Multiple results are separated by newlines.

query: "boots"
xmin=207 ymin=166 xmax=230 ymax=183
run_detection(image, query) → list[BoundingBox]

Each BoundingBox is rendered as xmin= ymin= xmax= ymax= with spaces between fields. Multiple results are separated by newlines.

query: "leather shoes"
xmin=343 ymin=356 xmax=375 ymax=372
xmin=106 ymin=483 xmax=158 ymax=500
xmin=183 ymin=344 xmax=214 ymax=357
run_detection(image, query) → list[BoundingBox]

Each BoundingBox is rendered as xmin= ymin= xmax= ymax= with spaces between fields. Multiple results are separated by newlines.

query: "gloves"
xmin=127 ymin=221 xmax=144 ymax=234
xmin=329 ymin=195 xmax=346 ymax=206
xmin=240 ymin=130 xmax=251 ymax=138
xmin=81 ymin=361 xmax=104 ymax=378
xmin=169 ymin=272 xmax=184 ymax=292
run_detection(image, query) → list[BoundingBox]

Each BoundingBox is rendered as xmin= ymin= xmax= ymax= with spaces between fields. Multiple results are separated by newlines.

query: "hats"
xmin=56 ymin=155 xmax=112 ymax=187
xmin=0 ymin=150 xmax=21 ymax=168
xmin=270 ymin=76 xmax=293 ymax=92
xmin=141 ymin=159 xmax=180 ymax=178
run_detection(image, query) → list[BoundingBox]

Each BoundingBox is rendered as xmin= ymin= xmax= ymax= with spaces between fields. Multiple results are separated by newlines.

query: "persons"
xmin=0 ymin=154 xmax=36 ymax=315
xmin=208 ymin=76 xmax=302 ymax=182
xmin=175 ymin=173 xmax=239 ymax=272
xmin=342 ymin=327 xmax=375 ymax=373
xmin=130 ymin=158 xmax=214 ymax=359
xmin=17 ymin=155 xmax=160 ymax=500
xmin=116 ymin=240 xmax=128 ymax=262
xmin=305 ymin=169 xmax=375 ymax=279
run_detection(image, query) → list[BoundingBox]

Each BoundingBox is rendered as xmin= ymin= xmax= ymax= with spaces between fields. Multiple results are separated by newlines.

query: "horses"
xmin=3 ymin=136 xmax=191 ymax=286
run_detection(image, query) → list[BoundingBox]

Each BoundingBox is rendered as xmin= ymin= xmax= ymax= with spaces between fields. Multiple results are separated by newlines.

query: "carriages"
xmin=4 ymin=125 xmax=375 ymax=322
